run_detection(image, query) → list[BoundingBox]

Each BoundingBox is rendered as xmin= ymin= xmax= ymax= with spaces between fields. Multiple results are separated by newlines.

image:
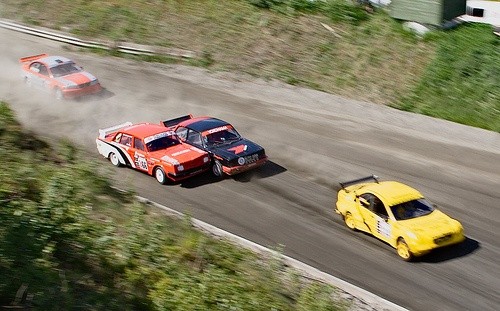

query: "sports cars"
xmin=163 ymin=115 xmax=268 ymax=175
xmin=96 ymin=121 xmax=214 ymax=185
xmin=337 ymin=176 xmax=464 ymax=260
xmin=21 ymin=54 xmax=102 ymax=101
